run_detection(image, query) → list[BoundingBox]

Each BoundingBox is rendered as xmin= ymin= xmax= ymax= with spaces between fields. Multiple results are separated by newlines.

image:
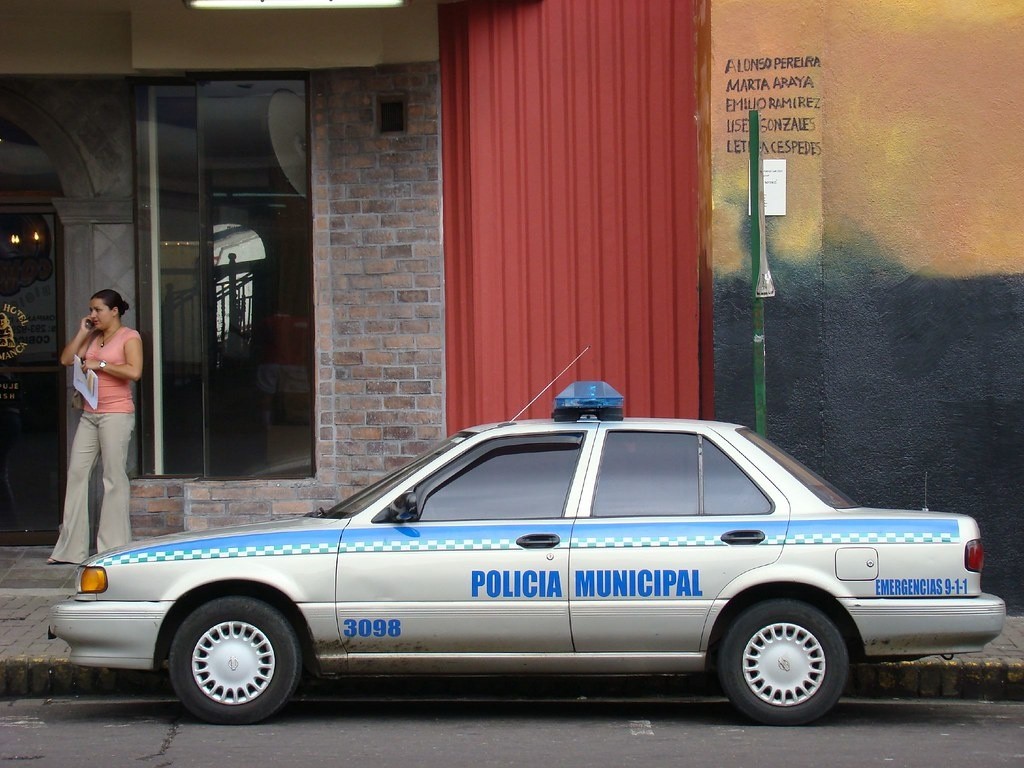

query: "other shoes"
xmin=46 ymin=558 xmax=60 ymax=564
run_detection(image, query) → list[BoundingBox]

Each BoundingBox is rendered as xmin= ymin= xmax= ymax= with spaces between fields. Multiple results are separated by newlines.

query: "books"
xmin=73 ymin=354 xmax=99 ymax=410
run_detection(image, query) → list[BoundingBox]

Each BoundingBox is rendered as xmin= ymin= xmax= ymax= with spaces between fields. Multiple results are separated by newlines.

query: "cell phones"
xmin=86 ymin=321 xmax=95 ymax=329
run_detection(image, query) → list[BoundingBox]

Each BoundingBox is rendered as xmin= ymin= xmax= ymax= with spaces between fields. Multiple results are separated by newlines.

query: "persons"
xmin=46 ymin=288 xmax=142 ymax=565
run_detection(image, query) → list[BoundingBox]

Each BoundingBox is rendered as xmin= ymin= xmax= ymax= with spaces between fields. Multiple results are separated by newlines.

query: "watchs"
xmin=99 ymin=360 xmax=108 ymax=373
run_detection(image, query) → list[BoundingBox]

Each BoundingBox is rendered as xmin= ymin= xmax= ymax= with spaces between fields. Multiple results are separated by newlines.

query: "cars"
xmin=46 ymin=379 xmax=1008 ymax=725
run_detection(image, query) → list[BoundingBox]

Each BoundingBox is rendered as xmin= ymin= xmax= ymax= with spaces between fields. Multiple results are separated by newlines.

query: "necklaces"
xmin=101 ymin=324 xmax=122 ymax=347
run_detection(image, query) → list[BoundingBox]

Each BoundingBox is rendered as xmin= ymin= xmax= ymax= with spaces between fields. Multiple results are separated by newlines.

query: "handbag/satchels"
xmin=72 ymin=331 xmax=101 ymax=409
